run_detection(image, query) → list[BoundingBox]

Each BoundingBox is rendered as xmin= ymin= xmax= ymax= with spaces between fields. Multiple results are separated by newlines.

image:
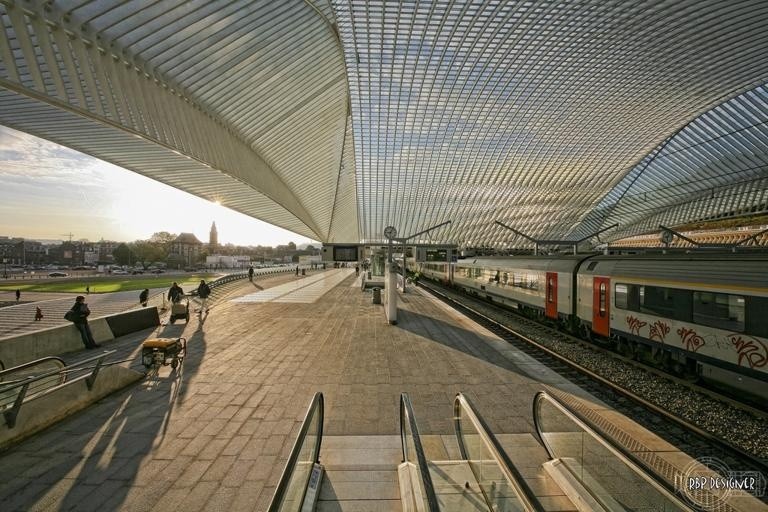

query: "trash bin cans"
xmin=302 ymin=269 xmax=305 ymax=275
xmin=372 ymin=287 xmax=381 ymax=304
xmin=368 ymin=272 xmax=371 ymax=279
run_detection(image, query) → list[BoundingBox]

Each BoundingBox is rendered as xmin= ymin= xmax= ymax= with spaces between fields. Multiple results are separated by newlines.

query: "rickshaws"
xmin=169 ymin=295 xmax=191 ymax=324
xmin=140 ymin=338 xmax=185 ymax=373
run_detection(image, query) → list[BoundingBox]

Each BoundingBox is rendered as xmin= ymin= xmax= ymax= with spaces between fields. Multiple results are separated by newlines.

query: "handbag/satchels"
xmin=64 ymin=311 xmax=74 ymax=321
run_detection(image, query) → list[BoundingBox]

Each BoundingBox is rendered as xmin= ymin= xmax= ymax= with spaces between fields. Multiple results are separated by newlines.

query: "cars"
xmin=48 ymin=272 xmax=68 ymax=277
xmin=109 ymin=264 xmax=144 ymax=275
xmin=150 ymin=268 xmax=166 ymax=273
xmin=58 ymin=266 xmax=68 ymax=270
xmin=72 ymin=265 xmax=96 ymax=270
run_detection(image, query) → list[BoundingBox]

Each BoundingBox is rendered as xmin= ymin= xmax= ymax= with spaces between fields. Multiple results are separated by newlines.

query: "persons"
xmin=35 ymin=306 xmax=41 ymax=321
xmin=249 ymin=267 xmax=254 ymax=282
xmin=198 ymin=279 xmax=210 ymax=313
xmin=168 ymin=282 xmax=184 ymax=305
xmin=140 ymin=289 xmax=149 ymax=307
xmin=295 ymin=266 xmax=298 ymax=276
xmin=72 ymin=296 xmax=99 ymax=349
xmin=16 ymin=289 xmax=20 ymax=301
xmin=356 ymin=265 xmax=359 ymax=276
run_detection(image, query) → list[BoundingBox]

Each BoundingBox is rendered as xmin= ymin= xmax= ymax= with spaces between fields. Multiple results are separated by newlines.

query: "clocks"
xmin=659 ymin=230 xmax=673 ymax=243
xmin=384 ymin=226 xmax=397 ymax=240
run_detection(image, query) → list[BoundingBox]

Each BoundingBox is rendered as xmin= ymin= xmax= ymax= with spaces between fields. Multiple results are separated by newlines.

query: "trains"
xmin=382 ymin=245 xmax=768 ymax=408
